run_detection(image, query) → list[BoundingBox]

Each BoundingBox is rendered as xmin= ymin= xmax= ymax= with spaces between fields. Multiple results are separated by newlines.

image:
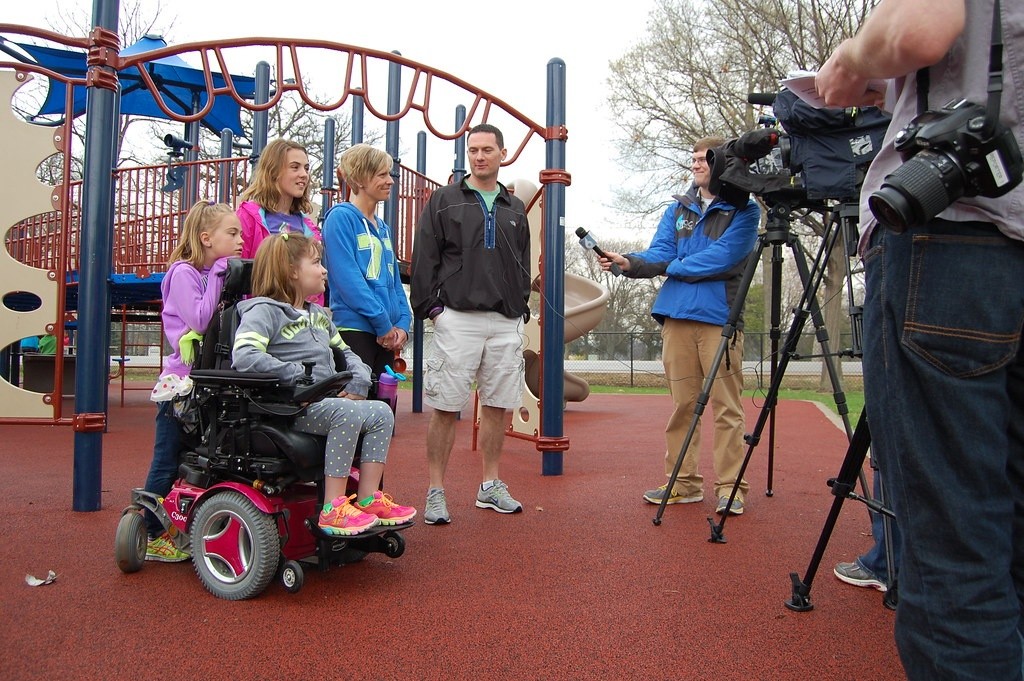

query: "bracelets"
xmin=429 ymin=307 xmax=443 ymax=317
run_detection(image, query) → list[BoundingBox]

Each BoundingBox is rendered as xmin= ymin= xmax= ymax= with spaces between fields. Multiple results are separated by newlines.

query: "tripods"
xmin=650 ymin=211 xmax=873 ymax=526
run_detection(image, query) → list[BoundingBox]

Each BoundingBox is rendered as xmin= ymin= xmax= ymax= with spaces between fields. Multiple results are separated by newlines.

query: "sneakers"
xmin=715 ymin=494 xmax=743 ymax=515
xmin=424 ymin=488 xmax=451 ymax=524
xmin=643 ymin=481 xmax=704 ymax=504
xmin=354 ymin=490 xmax=417 ymax=526
xmin=144 ymin=530 xmax=190 ymax=563
xmin=834 ymin=561 xmax=887 ymax=592
xmin=318 ymin=494 xmax=379 ymax=536
xmin=476 ymin=478 xmax=522 ymax=514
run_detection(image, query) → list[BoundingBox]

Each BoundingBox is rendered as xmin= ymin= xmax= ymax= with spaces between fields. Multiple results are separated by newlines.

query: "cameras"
xmin=868 ymin=98 xmax=1024 ymax=234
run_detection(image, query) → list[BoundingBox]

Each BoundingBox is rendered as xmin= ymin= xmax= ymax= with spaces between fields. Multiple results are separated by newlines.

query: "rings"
xmin=402 ymin=344 xmax=403 ymax=347
xmin=383 ymin=342 xmax=388 ymax=346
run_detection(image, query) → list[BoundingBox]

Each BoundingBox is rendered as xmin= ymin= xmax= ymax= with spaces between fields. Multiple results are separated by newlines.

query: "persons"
xmin=410 ymin=123 xmax=530 ymax=526
xmin=598 ymin=138 xmax=761 ymax=515
xmin=136 ymin=139 xmax=416 ymax=563
xmin=814 ymin=0 xmax=1024 ymax=681
xmin=39 ymin=330 xmax=69 ymax=355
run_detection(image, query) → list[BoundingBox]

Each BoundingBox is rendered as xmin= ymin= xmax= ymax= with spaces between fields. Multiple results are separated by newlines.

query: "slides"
xmin=525 ymin=262 xmax=610 ymax=409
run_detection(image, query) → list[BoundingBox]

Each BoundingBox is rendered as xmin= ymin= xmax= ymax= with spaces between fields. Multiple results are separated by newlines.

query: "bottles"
xmin=377 ymin=364 xmax=406 ymax=412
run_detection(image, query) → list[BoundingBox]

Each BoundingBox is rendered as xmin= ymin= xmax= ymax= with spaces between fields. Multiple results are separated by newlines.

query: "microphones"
xmin=575 ymin=227 xmax=624 ymax=277
xmin=488 ymin=212 xmax=492 ymax=218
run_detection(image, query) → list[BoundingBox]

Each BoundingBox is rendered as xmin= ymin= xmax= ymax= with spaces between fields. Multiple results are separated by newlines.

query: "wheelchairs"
xmin=114 ymin=257 xmax=416 ymax=603
